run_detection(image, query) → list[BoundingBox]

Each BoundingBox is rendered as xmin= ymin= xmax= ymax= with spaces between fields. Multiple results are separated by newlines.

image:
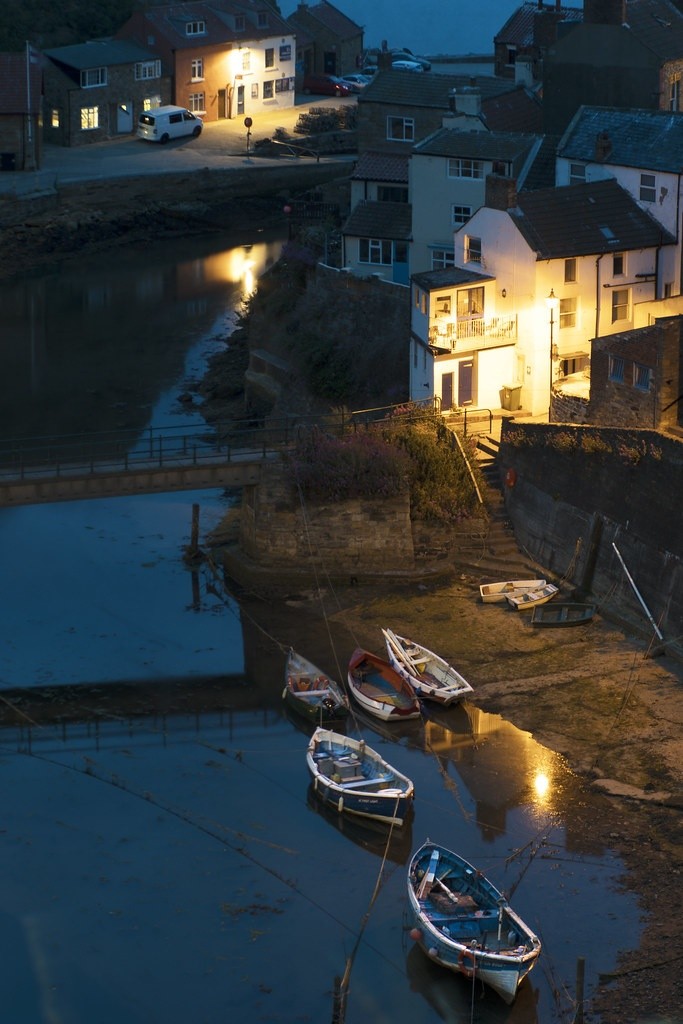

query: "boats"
xmin=307 ymin=726 xmax=414 ymax=829
xmin=531 ymin=603 xmax=596 ymax=627
xmin=407 ymin=837 xmax=541 ymax=1006
xmin=348 ymin=647 xmax=424 ymax=722
xmin=479 ymin=579 xmax=546 ymax=603
xmin=281 ymin=650 xmax=349 ymax=724
xmin=379 ymin=625 xmax=475 ymax=706
xmin=505 ymin=584 xmax=559 ymax=611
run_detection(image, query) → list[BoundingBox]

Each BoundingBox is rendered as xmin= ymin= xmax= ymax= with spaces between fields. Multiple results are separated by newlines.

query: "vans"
xmin=136 ymin=105 xmax=203 ymax=144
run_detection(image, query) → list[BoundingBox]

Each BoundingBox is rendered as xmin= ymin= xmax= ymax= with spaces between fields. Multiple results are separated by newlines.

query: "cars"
xmin=341 ymin=74 xmax=368 ymax=93
xmin=392 ymin=52 xmax=431 ymax=71
xmin=359 ymin=65 xmax=378 ymax=80
xmin=392 ymin=61 xmax=424 ymax=74
xmin=303 ymin=72 xmax=353 ymax=97
